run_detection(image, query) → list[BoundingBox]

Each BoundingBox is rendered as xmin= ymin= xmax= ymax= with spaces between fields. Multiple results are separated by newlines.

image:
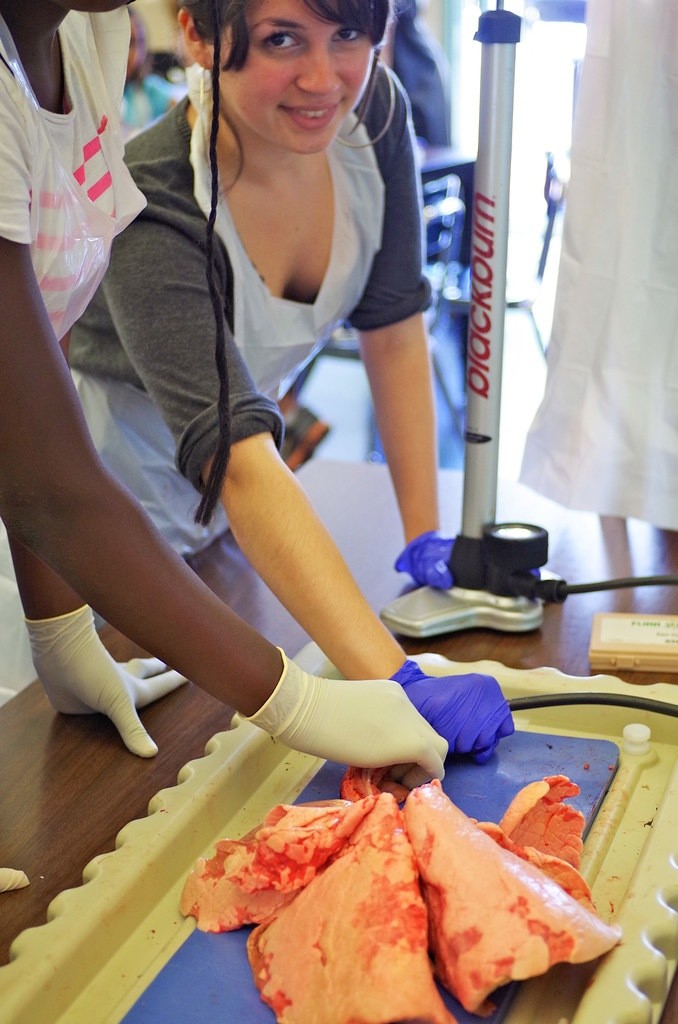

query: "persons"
xmin=368 ymin=0 xmax=466 ymax=467
xmin=0 ymin=1 xmax=454 ymax=793
xmin=118 ymin=4 xmax=186 ymax=135
xmin=60 ymin=1 xmax=512 ymax=760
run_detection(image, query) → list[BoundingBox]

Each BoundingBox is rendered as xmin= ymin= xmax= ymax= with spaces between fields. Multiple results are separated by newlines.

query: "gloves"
xmin=388 ymin=658 xmax=515 ymax=766
xmin=395 ymin=530 xmax=541 ymax=591
xmin=235 ymin=646 xmax=448 ymax=781
xmin=24 ymin=604 xmax=189 ymax=757
